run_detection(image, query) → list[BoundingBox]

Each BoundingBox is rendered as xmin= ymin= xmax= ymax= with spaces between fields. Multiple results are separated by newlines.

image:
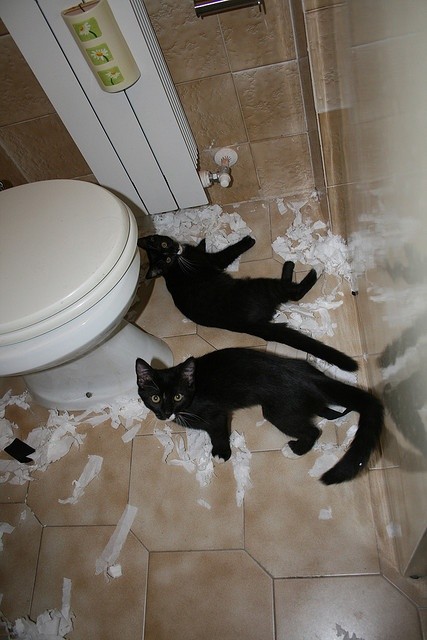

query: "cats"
xmin=134 ymin=348 xmax=386 ymax=486
xmin=137 ymin=233 xmax=362 ymax=373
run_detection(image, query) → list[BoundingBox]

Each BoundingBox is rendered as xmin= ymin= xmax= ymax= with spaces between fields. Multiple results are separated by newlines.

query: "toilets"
xmin=0 ymin=180 xmax=174 ymax=413
